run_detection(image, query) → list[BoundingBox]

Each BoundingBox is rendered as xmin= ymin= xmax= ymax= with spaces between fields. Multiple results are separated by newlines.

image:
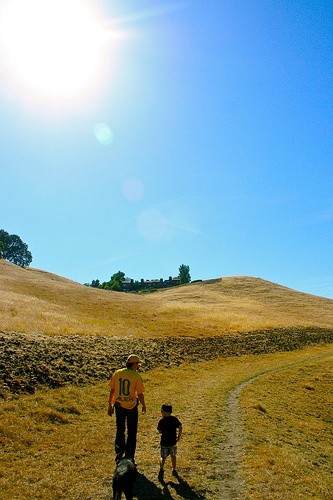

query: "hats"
xmin=126 ymin=354 xmax=143 ymax=363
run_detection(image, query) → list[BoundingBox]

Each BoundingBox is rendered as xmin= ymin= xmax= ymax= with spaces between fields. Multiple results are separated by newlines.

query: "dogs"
xmin=112 ymin=456 xmax=137 ymax=500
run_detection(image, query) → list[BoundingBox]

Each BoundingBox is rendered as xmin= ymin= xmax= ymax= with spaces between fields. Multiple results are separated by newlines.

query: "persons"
xmin=108 ymin=354 xmax=146 ymax=464
xmin=157 ymin=403 xmax=182 ymax=480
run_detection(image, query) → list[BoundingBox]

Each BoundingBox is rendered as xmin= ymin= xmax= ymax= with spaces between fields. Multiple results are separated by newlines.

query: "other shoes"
xmin=158 ymin=468 xmax=164 ymax=480
xmin=115 ymin=453 xmax=123 ymax=466
xmin=172 ymin=470 xmax=178 ymax=476
